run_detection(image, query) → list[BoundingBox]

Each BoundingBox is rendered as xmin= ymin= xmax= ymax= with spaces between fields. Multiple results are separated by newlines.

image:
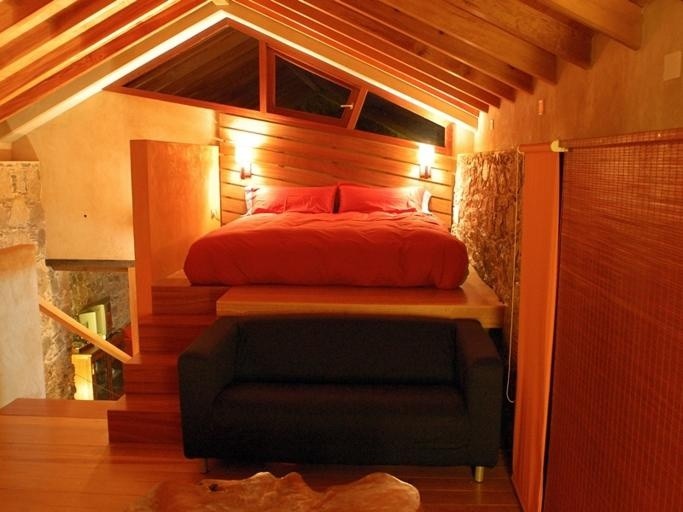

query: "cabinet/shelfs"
xmin=71 ymin=331 xmax=124 ymax=400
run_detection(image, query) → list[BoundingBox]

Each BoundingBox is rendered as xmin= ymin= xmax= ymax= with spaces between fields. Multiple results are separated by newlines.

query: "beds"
xmin=183 ymin=183 xmax=468 ymax=290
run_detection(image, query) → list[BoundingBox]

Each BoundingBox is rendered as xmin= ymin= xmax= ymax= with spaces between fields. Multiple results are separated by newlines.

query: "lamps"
xmin=240 ymin=156 xmax=253 ymax=180
xmin=420 ymin=155 xmax=433 ymax=179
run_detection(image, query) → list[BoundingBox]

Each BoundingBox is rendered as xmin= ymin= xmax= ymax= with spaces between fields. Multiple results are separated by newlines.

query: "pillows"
xmin=246 ymin=184 xmax=434 ymax=216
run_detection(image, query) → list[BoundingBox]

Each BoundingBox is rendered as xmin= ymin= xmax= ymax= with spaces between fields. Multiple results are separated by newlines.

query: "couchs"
xmin=177 ymin=311 xmax=504 ymax=485
